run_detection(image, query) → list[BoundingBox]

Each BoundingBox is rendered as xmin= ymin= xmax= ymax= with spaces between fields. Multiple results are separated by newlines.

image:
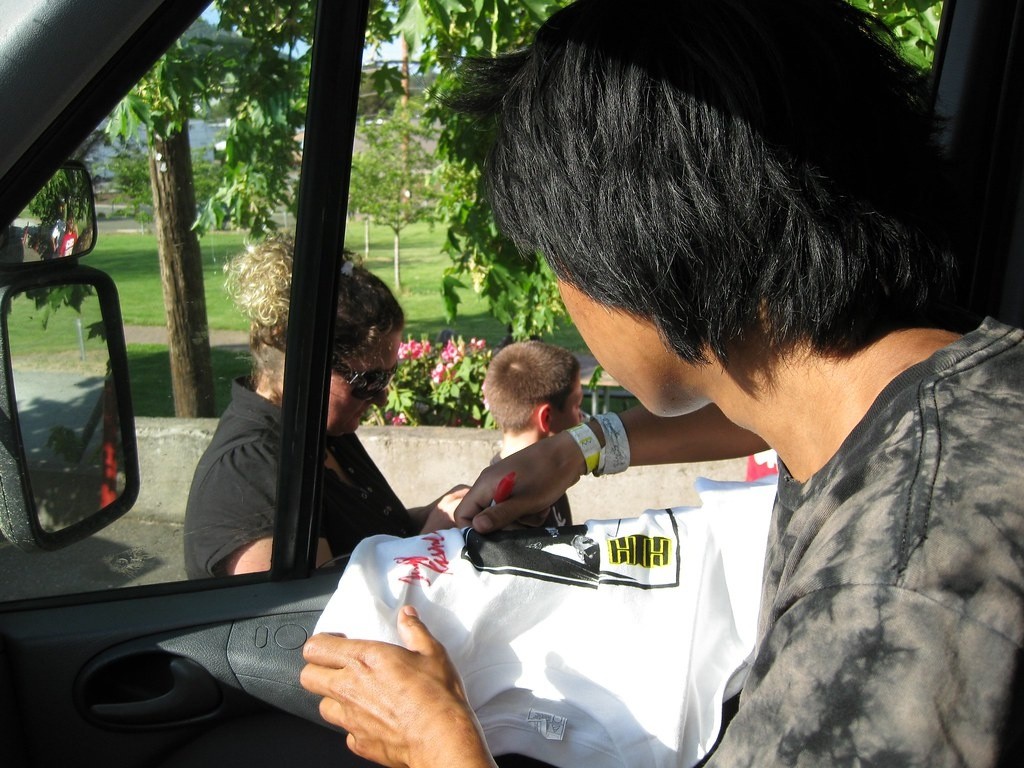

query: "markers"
xmin=490 ymin=472 xmax=516 ymax=507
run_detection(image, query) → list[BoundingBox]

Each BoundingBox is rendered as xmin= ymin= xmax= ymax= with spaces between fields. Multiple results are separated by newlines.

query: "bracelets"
xmin=566 ymin=411 xmax=631 ymax=476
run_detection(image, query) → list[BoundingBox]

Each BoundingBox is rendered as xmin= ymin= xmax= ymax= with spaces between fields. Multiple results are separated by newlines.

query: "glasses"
xmin=334 ymin=357 xmax=399 ymax=400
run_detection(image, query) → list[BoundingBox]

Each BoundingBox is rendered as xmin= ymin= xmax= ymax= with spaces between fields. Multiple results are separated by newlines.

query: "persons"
xmin=183 ymin=232 xmax=474 ymax=580
xmin=301 ymin=0 xmax=1024 ymax=768
xmin=484 ymin=340 xmax=583 ymax=527
xmin=22 ymin=207 xmax=79 ymax=258
